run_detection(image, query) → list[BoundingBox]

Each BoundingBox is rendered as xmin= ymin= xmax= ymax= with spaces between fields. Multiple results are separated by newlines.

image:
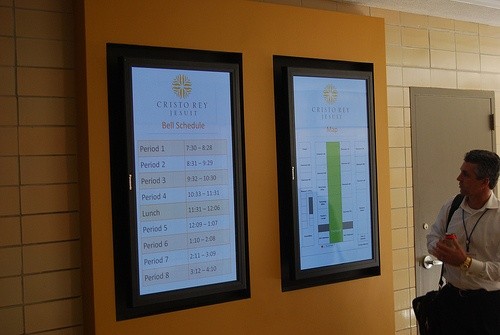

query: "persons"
xmin=426 ymin=150 xmax=496 ymax=334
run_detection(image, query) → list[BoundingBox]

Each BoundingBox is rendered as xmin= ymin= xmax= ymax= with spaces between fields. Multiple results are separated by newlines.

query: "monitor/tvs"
xmin=124 ymin=57 xmax=247 ymax=307
xmin=288 ymin=67 xmax=379 ymax=281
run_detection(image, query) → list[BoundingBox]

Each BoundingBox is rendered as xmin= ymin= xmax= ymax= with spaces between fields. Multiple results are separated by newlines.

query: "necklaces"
xmin=461 ymin=198 xmax=491 ymax=253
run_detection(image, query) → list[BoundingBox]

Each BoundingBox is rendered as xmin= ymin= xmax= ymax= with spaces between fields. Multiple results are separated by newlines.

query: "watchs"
xmin=459 ymin=256 xmax=474 ymax=269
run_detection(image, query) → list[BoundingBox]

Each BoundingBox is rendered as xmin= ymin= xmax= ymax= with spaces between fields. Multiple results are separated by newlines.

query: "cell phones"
xmin=440 ymin=239 xmax=455 ymax=249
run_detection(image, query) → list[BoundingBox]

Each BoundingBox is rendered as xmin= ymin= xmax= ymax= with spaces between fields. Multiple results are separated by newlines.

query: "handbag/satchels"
xmin=411 ymin=284 xmax=465 ymax=335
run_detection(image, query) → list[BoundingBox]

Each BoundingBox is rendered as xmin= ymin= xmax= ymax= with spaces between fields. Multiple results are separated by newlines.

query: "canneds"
xmin=444 ymin=233 xmax=457 ymax=248
